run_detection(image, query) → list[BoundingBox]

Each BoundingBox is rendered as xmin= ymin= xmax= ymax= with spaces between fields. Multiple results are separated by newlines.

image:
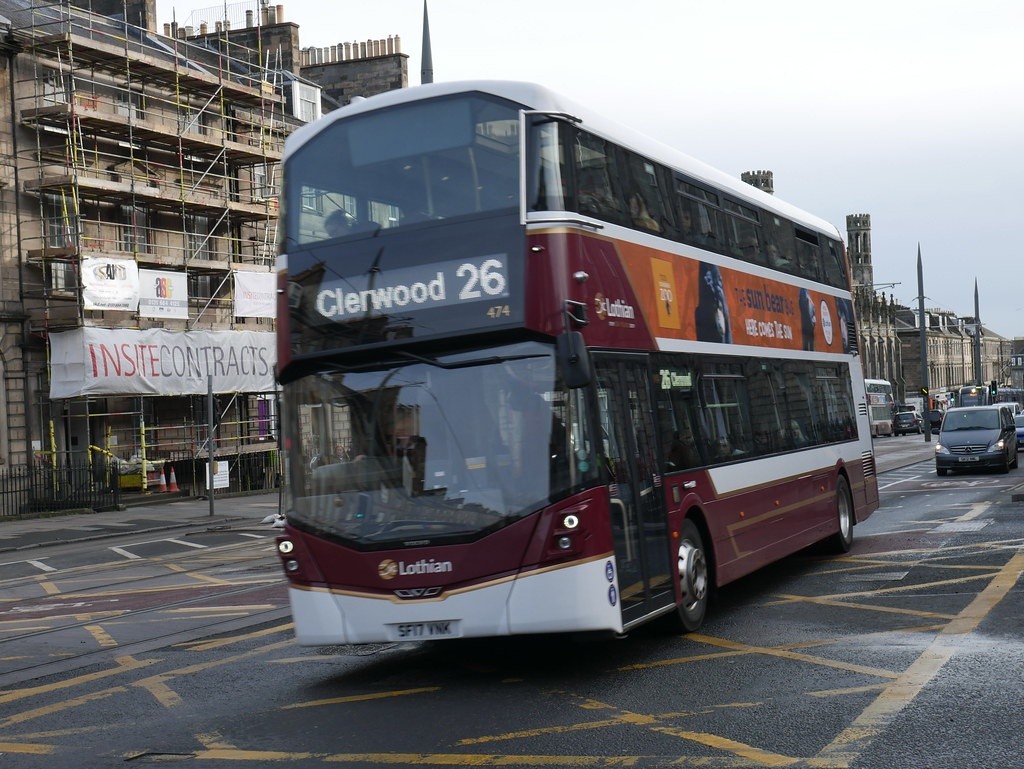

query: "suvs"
xmin=894 ymin=411 xmax=925 ymax=435
xmin=929 ymin=409 xmax=944 ymax=434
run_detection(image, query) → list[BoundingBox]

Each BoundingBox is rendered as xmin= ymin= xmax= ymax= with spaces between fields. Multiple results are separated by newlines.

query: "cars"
xmin=993 ymin=402 xmax=1024 ymax=448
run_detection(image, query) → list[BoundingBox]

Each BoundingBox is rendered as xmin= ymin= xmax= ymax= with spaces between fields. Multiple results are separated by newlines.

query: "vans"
xmin=932 ymin=406 xmax=1018 ymax=476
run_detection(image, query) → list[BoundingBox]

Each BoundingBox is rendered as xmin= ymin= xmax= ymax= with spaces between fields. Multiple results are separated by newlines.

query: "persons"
xmin=713 ymin=436 xmax=748 ymax=459
xmin=331 ymin=446 xmax=350 ymax=464
xmin=681 ymin=209 xmax=706 ymax=245
xmin=629 ymin=192 xmax=660 ymax=232
xmin=767 ymin=244 xmax=790 ymax=268
xmin=324 ymin=210 xmax=384 ymax=238
xmin=782 ymin=419 xmax=805 ymax=442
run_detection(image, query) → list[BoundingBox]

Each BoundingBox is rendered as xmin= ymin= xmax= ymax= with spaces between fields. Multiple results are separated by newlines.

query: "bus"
xmin=864 ymin=378 xmax=898 ymax=437
xmin=959 ymin=385 xmax=1024 ymax=409
xmin=272 ymin=81 xmax=879 ymax=647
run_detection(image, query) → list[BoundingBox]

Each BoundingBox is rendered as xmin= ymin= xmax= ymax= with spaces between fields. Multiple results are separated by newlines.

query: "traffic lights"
xmin=921 ymin=386 xmax=928 ymax=396
xmin=991 ymin=380 xmax=998 ymax=395
